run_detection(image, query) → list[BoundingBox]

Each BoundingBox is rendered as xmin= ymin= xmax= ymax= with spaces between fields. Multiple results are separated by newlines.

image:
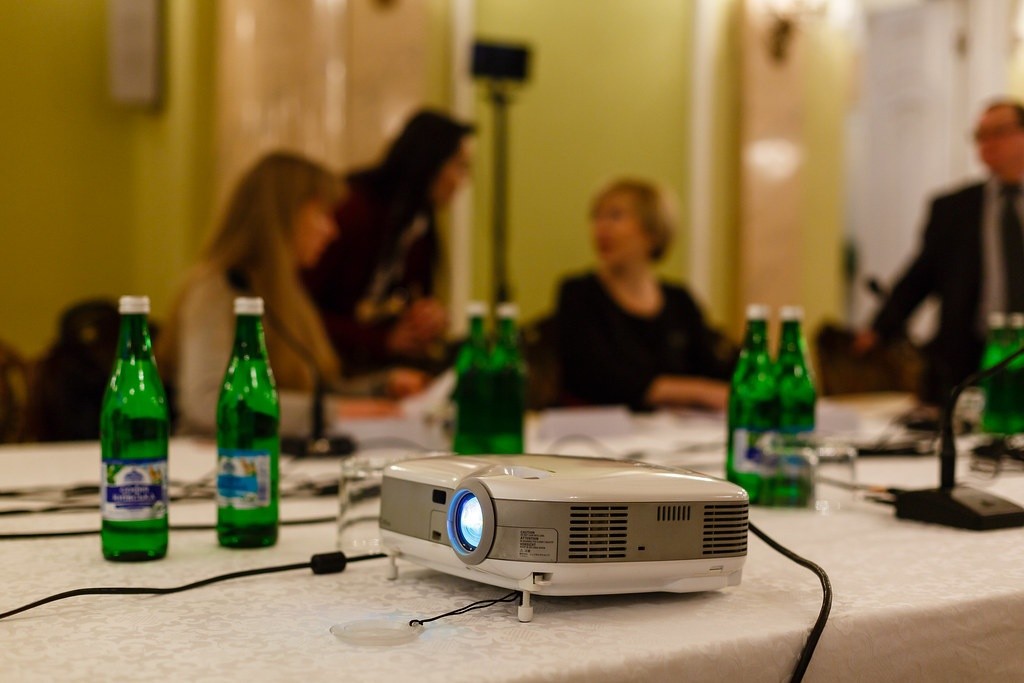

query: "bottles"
xmin=99 ymin=297 xmax=170 ymax=562
xmin=727 ymin=306 xmax=775 ymax=503
xmin=1005 ymin=314 xmax=1024 ymax=434
xmin=772 ymin=308 xmax=816 ymax=506
xmin=452 ymin=307 xmax=491 ymax=454
xmin=216 ymin=297 xmax=281 ymax=549
xmin=490 ymin=306 xmax=524 ymax=454
xmin=982 ymin=314 xmax=1005 ymax=433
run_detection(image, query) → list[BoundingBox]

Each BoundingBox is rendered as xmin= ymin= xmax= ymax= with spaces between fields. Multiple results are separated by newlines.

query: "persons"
xmin=520 ymin=179 xmax=742 ymax=416
xmin=302 ymin=110 xmax=479 ymax=377
xmin=849 ymin=102 xmax=1024 ymax=416
xmin=157 ymin=147 xmax=433 ymax=444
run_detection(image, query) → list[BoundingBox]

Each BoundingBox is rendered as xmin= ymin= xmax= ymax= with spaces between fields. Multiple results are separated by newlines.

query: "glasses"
xmin=975 ymin=123 xmax=1015 ymax=135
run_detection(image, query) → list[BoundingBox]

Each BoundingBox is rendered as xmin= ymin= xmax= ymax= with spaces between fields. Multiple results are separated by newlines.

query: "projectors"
xmin=379 ymin=455 xmax=749 ymax=624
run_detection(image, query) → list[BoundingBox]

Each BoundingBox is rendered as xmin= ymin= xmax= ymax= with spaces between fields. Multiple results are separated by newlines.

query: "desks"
xmin=0 ymin=387 xmax=1024 ymax=683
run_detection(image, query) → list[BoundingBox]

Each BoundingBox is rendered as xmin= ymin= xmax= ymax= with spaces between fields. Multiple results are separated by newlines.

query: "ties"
xmin=997 ymin=178 xmax=1023 ymax=314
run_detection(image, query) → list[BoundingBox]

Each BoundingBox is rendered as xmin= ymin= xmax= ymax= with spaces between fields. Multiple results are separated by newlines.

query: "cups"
xmin=812 ymin=446 xmax=858 ymax=514
xmin=338 ymin=457 xmax=385 ymax=555
